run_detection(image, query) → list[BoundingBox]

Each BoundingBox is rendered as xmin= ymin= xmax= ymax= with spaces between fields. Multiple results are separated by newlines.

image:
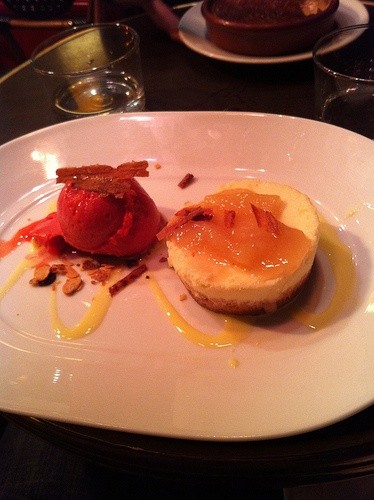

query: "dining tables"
xmin=0 ymin=0 xmax=374 ymax=500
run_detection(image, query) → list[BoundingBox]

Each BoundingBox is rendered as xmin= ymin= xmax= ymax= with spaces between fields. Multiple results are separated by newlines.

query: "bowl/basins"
xmin=201 ymin=0 xmax=340 ymax=56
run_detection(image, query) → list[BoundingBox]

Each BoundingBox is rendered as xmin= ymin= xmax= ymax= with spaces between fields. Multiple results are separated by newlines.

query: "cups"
xmin=32 ymin=23 xmax=145 ymax=123
xmin=313 ymin=24 xmax=374 ymax=142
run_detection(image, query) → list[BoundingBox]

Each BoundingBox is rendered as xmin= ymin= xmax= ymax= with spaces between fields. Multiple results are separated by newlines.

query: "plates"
xmin=0 ymin=111 xmax=373 ymax=442
xmin=178 ymin=0 xmax=368 ymax=64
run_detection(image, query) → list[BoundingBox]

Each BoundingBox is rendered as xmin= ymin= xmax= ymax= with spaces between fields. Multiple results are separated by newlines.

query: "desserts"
xmin=52 ymin=160 xmax=162 ymax=259
xmin=166 ymin=180 xmax=320 ymax=314
